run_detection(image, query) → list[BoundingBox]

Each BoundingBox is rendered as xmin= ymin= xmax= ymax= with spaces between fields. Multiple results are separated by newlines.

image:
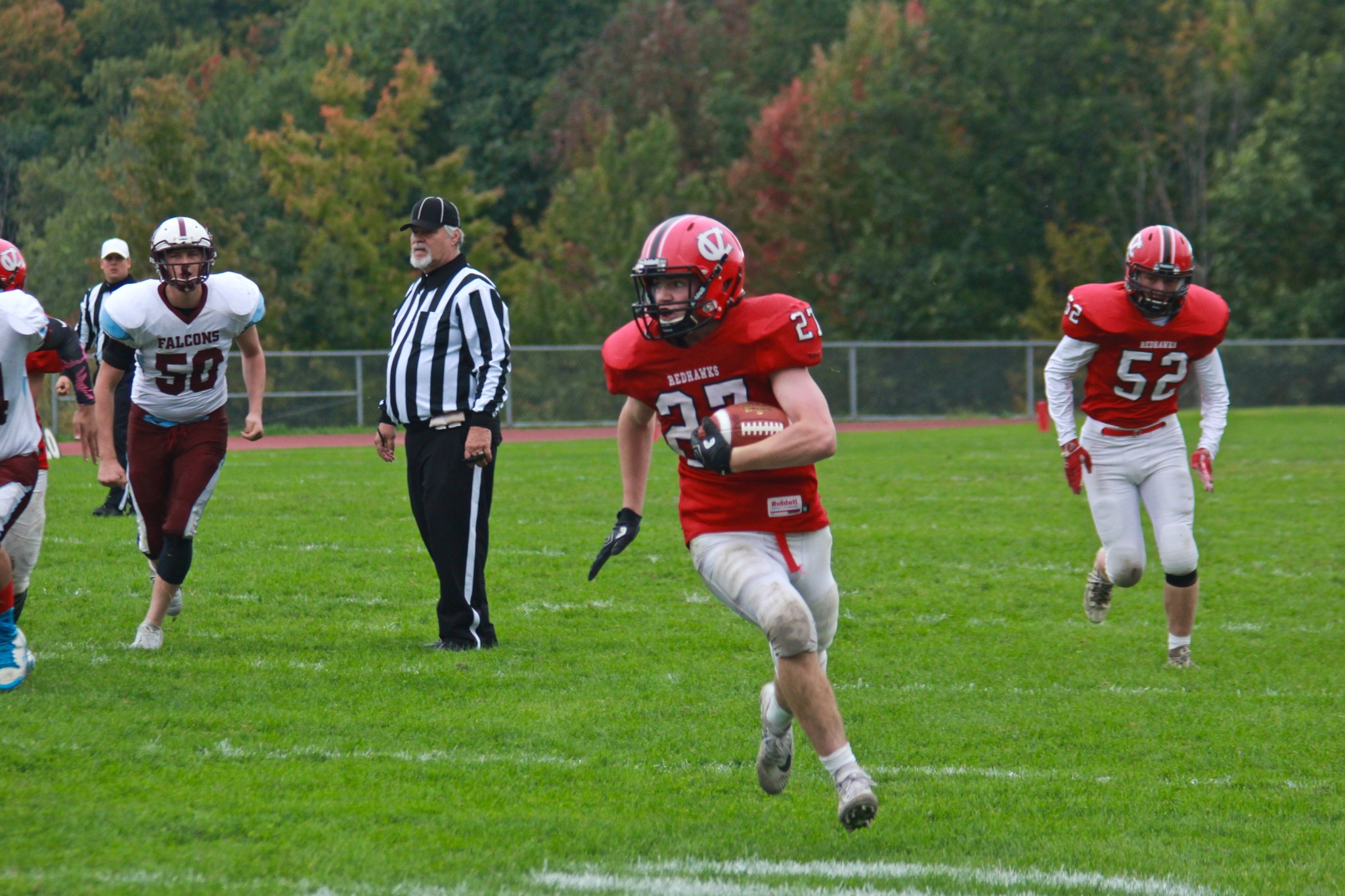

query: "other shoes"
xmin=437 ymin=640 xmax=470 ymax=652
xmin=425 ymin=639 xmax=442 ymax=647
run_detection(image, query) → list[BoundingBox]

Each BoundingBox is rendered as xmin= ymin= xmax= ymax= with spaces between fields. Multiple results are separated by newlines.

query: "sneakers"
xmin=91 ymin=496 xmax=124 ymax=514
xmin=1083 ymin=548 xmax=1115 ymax=624
xmin=833 ymin=761 xmax=879 ymax=830
xmin=760 ymin=683 xmax=794 ymax=793
xmin=1169 ymin=646 xmax=1191 ymax=672
xmin=0 ymin=617 xmax=34 ymax=689
xmin=129 ymin=622 xmax=163 ymax=650
xmin=148 ymin=559 xmax=181 ymax=617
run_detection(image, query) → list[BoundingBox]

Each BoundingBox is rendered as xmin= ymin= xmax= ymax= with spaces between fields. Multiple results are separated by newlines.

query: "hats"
xmin=399 ymin=196 xmax=460 ymax=231
xmin=101 ymin=237 xmax=129 ymax=259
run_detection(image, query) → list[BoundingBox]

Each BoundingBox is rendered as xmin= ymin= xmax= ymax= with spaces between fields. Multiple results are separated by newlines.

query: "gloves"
xmin=1191 ymin=449 xmax=1216 ymax=493
xmin=1060 ymin=439 xmax=1093 ymax=495
xmin=690 ymin=416 xmax=732 ymax=475
xmin=588 ymin=507 xmax=643 ymax=581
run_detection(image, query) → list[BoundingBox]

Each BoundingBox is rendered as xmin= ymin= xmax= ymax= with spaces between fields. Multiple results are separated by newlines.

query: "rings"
xmin=478 ymin=451 xmax=484 ymax=455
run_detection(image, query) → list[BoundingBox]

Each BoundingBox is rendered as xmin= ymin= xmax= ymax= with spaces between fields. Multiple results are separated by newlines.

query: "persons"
xmin=1043 ymin=224 xmax=1231 ymax=673
xmin=55 ymin=238 xmax=138 ymax=518
xmin=92 ymin=216 xmax=267 ymax=650
xmin=373 ymin=196 xmax=512 ymax=653
xmin=586 ymin=213 xmax=880 ymax=837
xmin=0 ymin=237 xmax=102 ymax=692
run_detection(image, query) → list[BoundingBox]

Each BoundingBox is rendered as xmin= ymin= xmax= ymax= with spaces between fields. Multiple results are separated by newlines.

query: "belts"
xmin=435 ymin=422 xmax=461 ymax=430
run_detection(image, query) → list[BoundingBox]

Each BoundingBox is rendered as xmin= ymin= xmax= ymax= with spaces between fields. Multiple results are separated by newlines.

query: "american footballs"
xmin=696 ymin=400 xmax=793 ymax=446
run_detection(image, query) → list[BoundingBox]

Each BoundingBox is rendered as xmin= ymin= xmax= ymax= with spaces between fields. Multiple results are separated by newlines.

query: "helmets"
xmin=633 ymin=213 xmax=745 ymax=345
xmin=1125 ymin=224 xmax=1194 ymax=319
xmin=0 ymin=238 xmax=27 ymax=290
xmin=149 ymin=216 xmax=217 ymax=291
